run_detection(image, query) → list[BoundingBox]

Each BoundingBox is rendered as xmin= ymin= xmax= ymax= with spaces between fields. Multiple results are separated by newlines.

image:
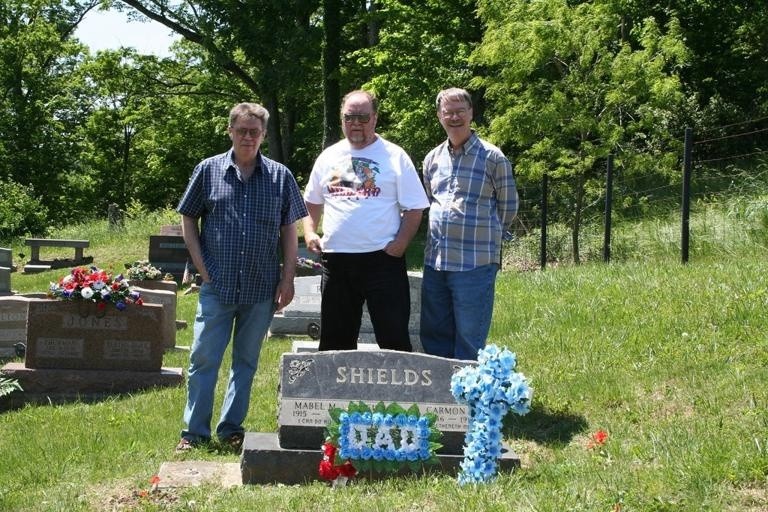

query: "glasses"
xmin=237 ymin=128 xmax=260 ymax=137
xmin=444 ymin=110 xmax=465 ymax=116
xmin=344 ymin=113 xmax=370 ymax=123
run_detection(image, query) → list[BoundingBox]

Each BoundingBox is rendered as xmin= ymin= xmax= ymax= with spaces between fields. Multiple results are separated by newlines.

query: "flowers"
xmin=296 ymin=256 xmax=322 ymax=275
xmin=124 ymin=262 xmax=174 ymax=281
xmin=46 ymin=265 xmax=143 ymax=319
xmin=450 ymin=344 xmax=535 ymax=486
xmin=319 ymin=401 xmax=444 ymax=480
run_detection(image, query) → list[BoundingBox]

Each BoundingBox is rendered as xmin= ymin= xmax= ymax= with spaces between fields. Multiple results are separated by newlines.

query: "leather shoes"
xmin=228 ymin=432 xmax=244 ymax=451
xmin=175 ymin=436 xmax=194 ymax=453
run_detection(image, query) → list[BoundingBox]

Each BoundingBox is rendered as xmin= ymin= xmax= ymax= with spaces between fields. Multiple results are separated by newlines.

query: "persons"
xmin=175 ymin=99 xmax=308 ymax=454
xmin=300 ymin=87 xmax=431 ymax=350
xmin=420 ymin=86 xmax=521 ymax=360
